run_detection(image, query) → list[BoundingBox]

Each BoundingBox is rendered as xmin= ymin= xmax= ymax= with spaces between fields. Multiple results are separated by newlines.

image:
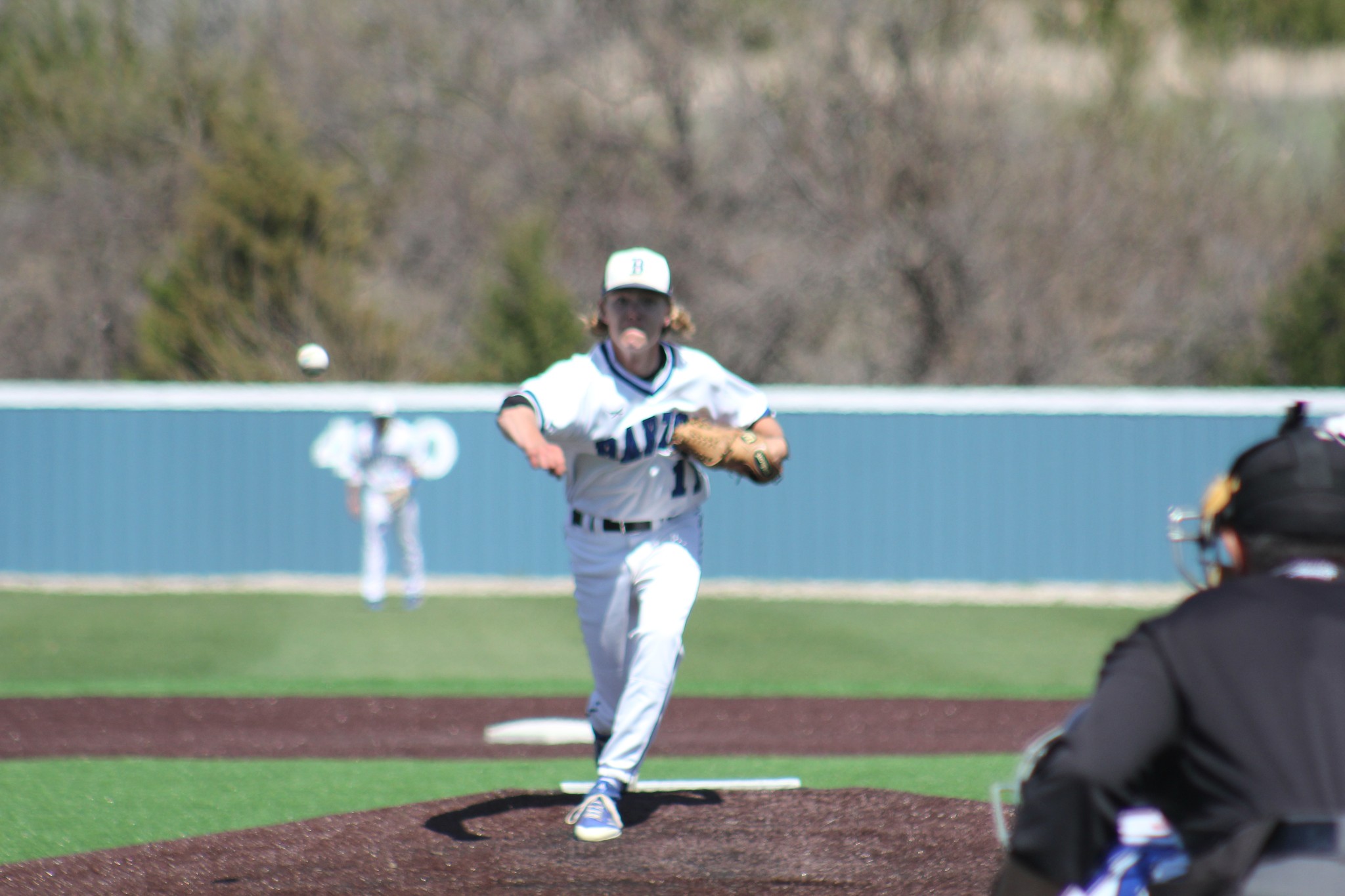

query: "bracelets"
xmin=406 ymin=479 xmax=419 ymax=493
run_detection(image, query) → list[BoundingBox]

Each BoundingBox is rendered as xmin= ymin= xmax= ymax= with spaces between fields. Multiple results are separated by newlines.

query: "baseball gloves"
xmin=671 ymin=415 xmax=783 ymax=486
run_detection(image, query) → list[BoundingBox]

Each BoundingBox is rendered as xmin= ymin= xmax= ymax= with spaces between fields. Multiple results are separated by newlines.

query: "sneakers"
xmin=564 ymin=776 xmax=627 ymax=842
xmin=595 ymin=739 xmax=607 ymax=764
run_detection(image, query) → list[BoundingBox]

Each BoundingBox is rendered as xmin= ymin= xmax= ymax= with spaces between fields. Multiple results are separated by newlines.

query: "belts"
xmin=573 ymin=511 xmax=671 ymax=532
xmin=1261 ymin=823 xmax=1336 ymax=856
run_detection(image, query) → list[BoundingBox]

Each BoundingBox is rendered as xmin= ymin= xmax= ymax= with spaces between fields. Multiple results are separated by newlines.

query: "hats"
xmin=374 ymin=402 xmax=394 ymax=419
xmin=1198 ymin=401 xmax=1345 ymax=551
xmin=602 ymin=247 xmax=674 ymax=297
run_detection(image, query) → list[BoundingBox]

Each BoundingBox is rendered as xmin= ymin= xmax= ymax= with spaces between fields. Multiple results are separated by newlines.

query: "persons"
xmin=497 ymin=249 xmax=786 ymax=841
xmin=347 ymin=402 xmax=423 ymax=613
xmin=985 ymin=402 xmax=1345 ymax=896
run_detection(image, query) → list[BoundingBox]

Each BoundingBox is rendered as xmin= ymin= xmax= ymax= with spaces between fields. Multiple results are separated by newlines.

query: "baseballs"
xmin=296 ymin=343 xmax=329 ymax=378
